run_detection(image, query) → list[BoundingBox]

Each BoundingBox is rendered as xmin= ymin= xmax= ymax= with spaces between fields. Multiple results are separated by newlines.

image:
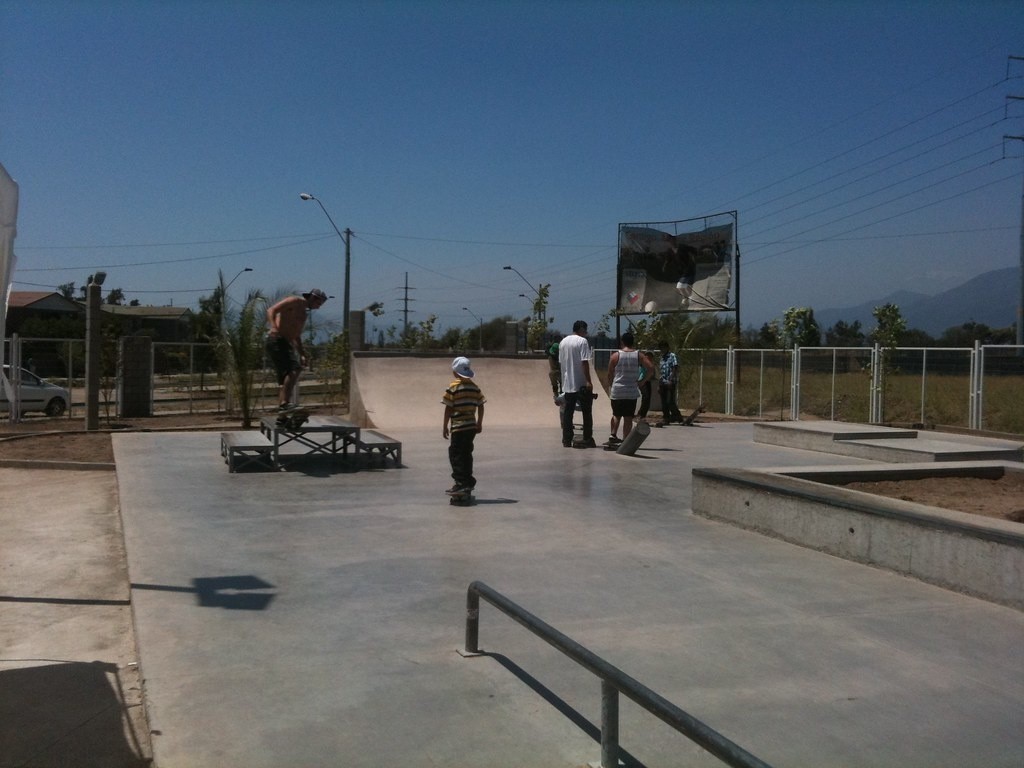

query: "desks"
xmin=261 ymin=414 xmax=361 ymax=472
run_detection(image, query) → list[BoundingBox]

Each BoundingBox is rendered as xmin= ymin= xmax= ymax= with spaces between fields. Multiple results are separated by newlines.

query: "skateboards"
xmin=446 ymin=486 xmax=472 ymax=505
xmin=571 ymin=434 xmax=587 ymax=448
xmin=682 ymin=402 xmax=708 ymax=426
xmin=277 ymin=405 xmax=310 ymax=418
xmin=601 ymin=439 xmax=621 ymax=451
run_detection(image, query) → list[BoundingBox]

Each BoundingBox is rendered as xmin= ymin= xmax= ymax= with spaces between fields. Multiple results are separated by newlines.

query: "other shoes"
xmin=563 ymin=439 xmax=572 ymax=447
xmin=670 ymin=414 xmax=683 ymax=423
xmin=445 ymin=484 xmax=464 ymax=493
xmin=660 ymin=420 xmax=669 ymax=426
xmin=554 ymin=395 xmax=560 ymax=406
xmin=681 ymin=295 xmax=693 ymax=306
xmin=275 ymin=416 xmax=290 ymax=426
xmin=464 ymin=477 xmax=477 ymax=491
xmin=278 ymin=402 xmax=305 ymax=414
xmin=585 ymin=438 xmax=596 ymax=448
xmin=609 ymin=434 xmax=623 ymax=443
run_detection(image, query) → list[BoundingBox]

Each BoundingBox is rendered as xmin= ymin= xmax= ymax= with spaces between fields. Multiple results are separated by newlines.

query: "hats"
xmin=549 ymin=343 xmax=559 ymax=355
xmin=452 ymin=357 xmax=474 ymax=378
xmin=302 ymin=288 xmax=328 ymax=302
xmin=644 ymin=350 xmax=655 ymax=356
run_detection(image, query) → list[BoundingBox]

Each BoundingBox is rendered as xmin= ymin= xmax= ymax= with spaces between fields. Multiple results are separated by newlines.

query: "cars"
xmin=0 ymin=365 xmax=70 ymax=417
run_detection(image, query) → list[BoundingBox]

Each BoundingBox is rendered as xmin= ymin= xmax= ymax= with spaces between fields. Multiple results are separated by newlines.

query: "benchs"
xmin=220 ymin=431 xmax=273 ymax=476
xmin=342 ymin=431 xmax=401 ymax=469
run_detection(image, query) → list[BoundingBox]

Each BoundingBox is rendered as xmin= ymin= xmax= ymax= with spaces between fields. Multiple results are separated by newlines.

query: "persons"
xmin=441 ymin=356 xmax=487 ymax=493
xmin=264 ymin=288 xmax=327 ymax=426
xmin=657 ymin=339 xmax=684 ymax=425
xmin=607 ymin=332 xmax=653 ymax=443
xmin=638 ymin=351 xmax=656 ymax=418
xmin=546 ymin=336 xmax=563 ymax=398
xmin=559 ymin=321 xmax=597 ymax=448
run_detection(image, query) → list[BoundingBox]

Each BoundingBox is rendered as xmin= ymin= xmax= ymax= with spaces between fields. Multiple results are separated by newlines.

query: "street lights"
xmin=299 ymin=193 xmax=350 ymax=394
xmin=462 ymin=307 xmax=483 ymax=351
xmin=503 ymin=266 xmax=542 ymax=350
